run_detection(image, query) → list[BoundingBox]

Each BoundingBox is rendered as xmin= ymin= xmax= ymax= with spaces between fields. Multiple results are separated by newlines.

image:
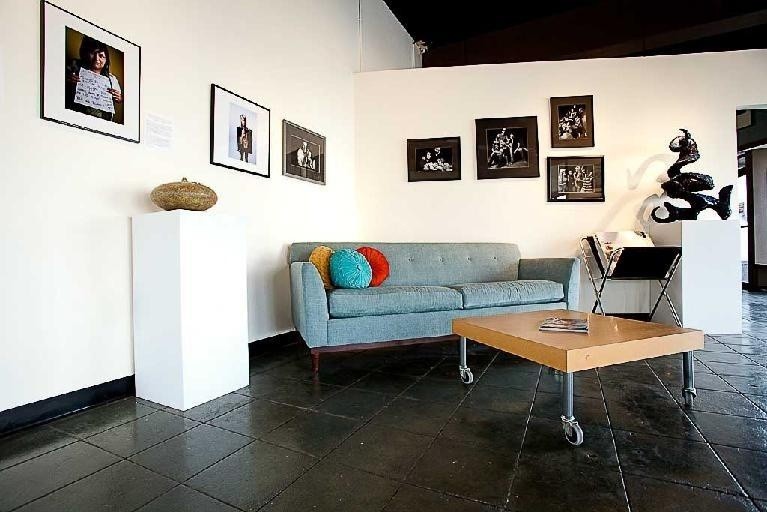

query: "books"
xmin=540 ymin=316 xmax=590 ymax=334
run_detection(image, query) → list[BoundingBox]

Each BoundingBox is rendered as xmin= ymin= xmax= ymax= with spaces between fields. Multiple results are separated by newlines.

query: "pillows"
xmin=307 ymin=247 xmax=390 ymax=291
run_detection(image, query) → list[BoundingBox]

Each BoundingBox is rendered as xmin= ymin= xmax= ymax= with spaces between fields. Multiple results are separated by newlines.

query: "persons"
xmin=559 ymin=104 xmax=587 ymax=139
xmin=67 ymin=35 xmax=123 ymax=121
xmin=488 ymin=128 xmax=528 ymax=168
xmin=297 ymin=141 xmax=313 ymax=169
xmin=558 ymin=166 xmax=594 ymax=192
xmin=421 ymin=147 xmax=452 ymax=171
xmin=237 ymin=115 xmax=252 ymax=163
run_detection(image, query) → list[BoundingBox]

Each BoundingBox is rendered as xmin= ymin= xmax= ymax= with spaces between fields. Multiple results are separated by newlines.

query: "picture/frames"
xmin=407 ymin=136 xmax=462 ymax=183
xmin=39 ymin=0 xmax=144 ymax=143
xmin=210 ymin=83 xmax=272 ymax=178
xmin=547 ymin=155 xmax=605 ymax=202
xmin=550 ymin=94 xmax=595 ymax=148
xmin=475 ymin=116 xmax=540 ymax=180
xmin=281 ymin=119 xmax=329 ymax=186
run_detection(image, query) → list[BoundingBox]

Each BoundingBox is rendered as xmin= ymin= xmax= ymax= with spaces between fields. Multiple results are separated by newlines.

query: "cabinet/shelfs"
xmin=580 ymin=232 xmax=683 ymax=328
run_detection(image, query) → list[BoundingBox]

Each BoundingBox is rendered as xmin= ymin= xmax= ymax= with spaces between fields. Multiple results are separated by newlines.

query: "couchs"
xmin=287 ymin=242 xmax=581 ymax=376
